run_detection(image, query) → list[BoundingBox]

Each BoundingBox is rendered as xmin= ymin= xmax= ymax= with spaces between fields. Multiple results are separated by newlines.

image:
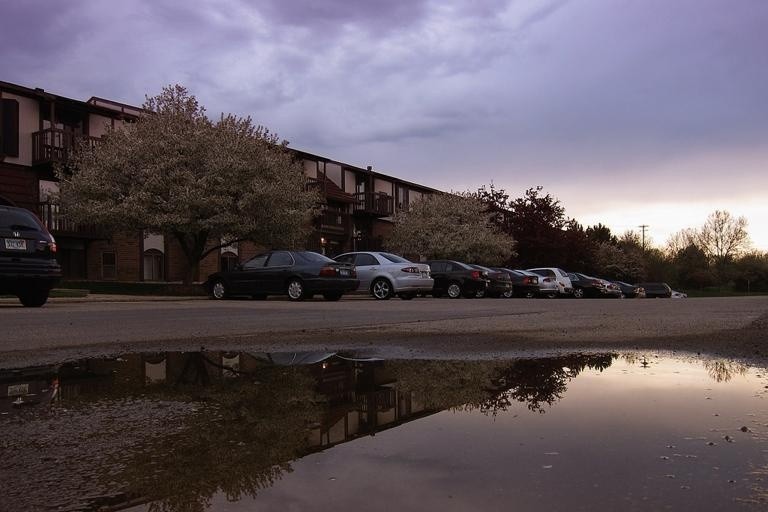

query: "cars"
xmin=565 ymin=272 xmax=607 ymax=299
xmin=331 ymin=250 xmax=434 ymax=301
xmin=466 ymin=263 xmax=514 ymax=299
xmin=592 ymin=277 xmax=688 ymax=299
xmin=0 ymin=205 xmax=66 ymax=309
xmin=419 ymin=258 xmax=492 ymax=299
xmin=487 ymin=267 xmax=539 ymax=299
xmin=514 ymin=269 xmax=560 ymax=299
xmin=522 ymin=266 xmax=575 ymax=298
xmin=200 ymin=248 xmax=361 ymax=301
